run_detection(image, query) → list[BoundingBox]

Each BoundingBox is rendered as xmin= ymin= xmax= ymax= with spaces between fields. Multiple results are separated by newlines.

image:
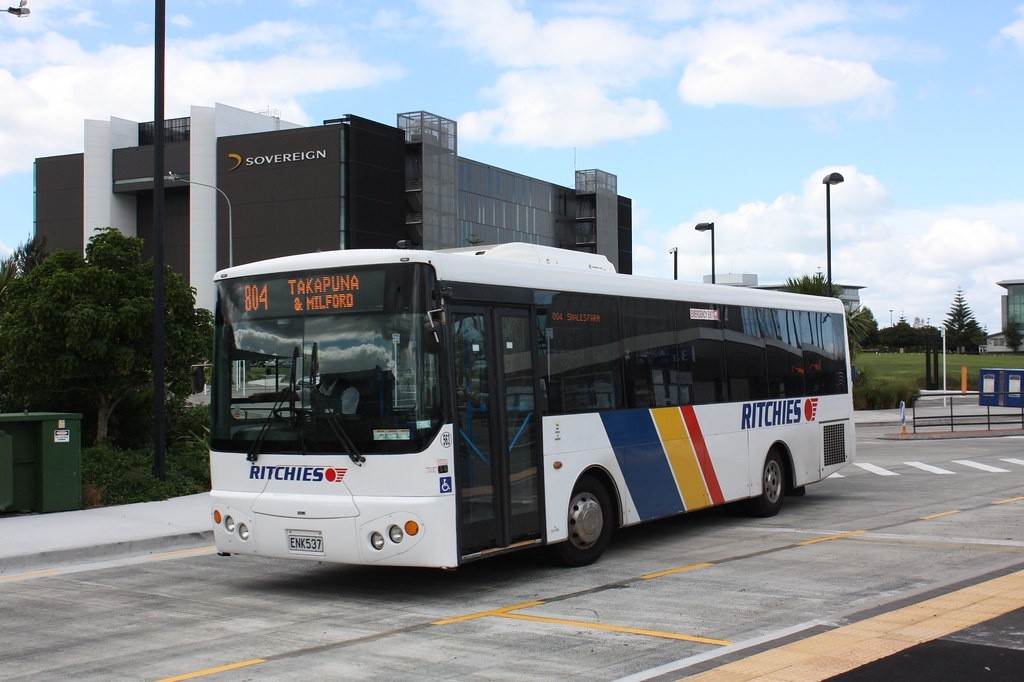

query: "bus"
xmin=209 ymin=241 xmax=857 ymax=569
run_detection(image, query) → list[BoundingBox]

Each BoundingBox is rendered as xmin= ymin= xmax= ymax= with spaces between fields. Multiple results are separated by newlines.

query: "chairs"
xmin=324 ymin=349 xmax=697 ymax=491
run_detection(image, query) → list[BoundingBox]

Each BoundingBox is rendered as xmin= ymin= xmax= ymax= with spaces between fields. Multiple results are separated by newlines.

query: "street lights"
xmin=670 ymin=247 xmax=678 ymax=279
xmin=822 ymin=173 xmax=844 ymax=296
xmin=695 ymin=222 xmax=717 ymax=285
xmin=164 ymin=175 xmax=232 ymax=268
